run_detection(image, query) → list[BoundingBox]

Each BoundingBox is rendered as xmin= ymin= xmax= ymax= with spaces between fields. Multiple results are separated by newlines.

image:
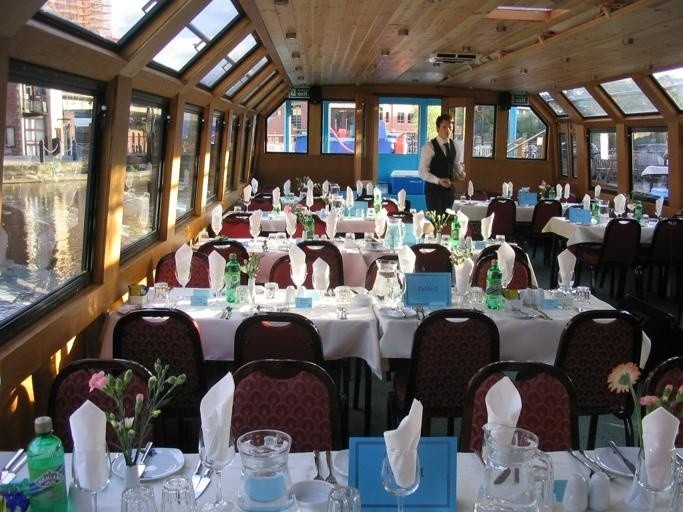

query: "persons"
xmin=418 ymin=114 xmax=467 ymax=215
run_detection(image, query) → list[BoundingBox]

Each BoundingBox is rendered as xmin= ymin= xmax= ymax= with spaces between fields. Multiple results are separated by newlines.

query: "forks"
xmin=312 ymin=448 xmax=324 ymax=480
xmin=579 ymin=449 xmax=615 ymax=480
xmin=325 ymin=449 xmax=337 ymax=484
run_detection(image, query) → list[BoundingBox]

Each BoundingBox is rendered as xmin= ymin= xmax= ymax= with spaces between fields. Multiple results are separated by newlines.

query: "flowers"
xmin=606 ymin=361 xmax=682 ymax=451
xmin=241 ymin=252 xmax=264 ymax=281
xmin=89 ymin=356 xmax=187 ymax=466
xmin=450 ymin=239 xmax=475 ymax=263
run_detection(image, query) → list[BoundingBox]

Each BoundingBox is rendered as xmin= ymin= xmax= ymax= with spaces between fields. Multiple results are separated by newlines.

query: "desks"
xmin=369 ymin=287 xmax=653 ymax=431
xmin=100 ymin=286 xmax=383 ymax=439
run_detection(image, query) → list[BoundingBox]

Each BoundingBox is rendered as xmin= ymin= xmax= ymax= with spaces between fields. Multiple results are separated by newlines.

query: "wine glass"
xmin=615 ymin=208 xmax=626 ymax=218
xmin=655 ymin=209 xmax=662 ymax=222
xmin=175 ymin=267 xmax=192 ymax=301
xmin=208 ymin=272 xmax=225 ymax=305
xmin=244 ymin=201 xmax=251 ymax=211
xmin=481 ymin=232 xmax=492 ymax=243
xmin=635 ymin=447 xmax=676 ymax=512
xmin=468 ymin=192 xmax=473 ymax=200
xmin=198 ymin=429 xmax=236 ymax=512
xmin=250 ymin=229 xmax=260 ymax=243
xmin=413 ymin=231 xmax=423 ymax=244
xmin=286 ymin=227 xmax=297 ymax=240
xmin=71 ymin=445 xmax=112 ymax=512
xmin=376 ymin=227 xmax=383 ymax=241
xmin=312 ymin=272 xmax=330 ymax=309
xmin=336 ymin=286 xmax=352 ymax=320
xmin=564 ymin=196 xmax=570 ymax=203
xmin=325 ymin=228 xmax=338 ymax=242
xmin=557 ymin=272 xmax=576 ymax=310
xmin=576 ymin=286 xmax=592 ymax=312
xmin=306 ymin=202 xmax=313 ymax=212
xmin=212 ymin=223 xmax=222 ymax=240
xmin=346 ymin=204 xmax=353 ymax=219
xmin=381 ymin=450 xmax=421 ymax=512
xmin=290 ymin=264 xmax=308 ymax=287
xmin=501 ymin=271 xmax=514 ymax=288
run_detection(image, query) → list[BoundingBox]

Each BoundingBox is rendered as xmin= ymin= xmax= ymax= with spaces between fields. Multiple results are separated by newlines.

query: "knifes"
xmin=608 ymin=441 xmax=636 ymax=475
xmin=192 ymin=459 xmax=202 ymax=488
xmin=0 ymin=448 xmax=24 ymax=478
xmin=3 ymin=455 xmax=28 ymax=485
xmin=138 ymin=441 xmax=154 ymax=477
xmin=195 ymin=468 xmax=213 ymax=498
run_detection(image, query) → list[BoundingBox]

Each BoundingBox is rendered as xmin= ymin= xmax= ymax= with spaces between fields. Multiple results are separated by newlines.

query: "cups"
xmin=268 ymin=233 xmax=278 ymax=251
xmin=345 ymin=233 xmax=356 ymax=245
xmin=468 ymin=287 xmax=484 ymax=313
xmin=367 ymin=208 xmax=376 ymax=220
xmin=356 ymin=208 xmax=365 ymax=218
xmin=354 ymin=294 xmax=372 ymax=306
xmin=328 ymin=486 xmax=362 ymax=512
xmin=162 ymin=475 xmax=197 ymax=512
xmin=153 ymin=282 xmax=169 ymax=307
xmin=121 ymin=485 xmax=158 ymax=511
xmin=364 ymin=232 xmax=374 ymax=245
xmin=128 ymin=284 xmax=148 ymax=303
xmin=292 ymin=479 xmax=336 ymax=512
xmin=277 ymin=232 xmax=286 ymax=251
xmin=265 ymin=281 xmax=280 ymax=299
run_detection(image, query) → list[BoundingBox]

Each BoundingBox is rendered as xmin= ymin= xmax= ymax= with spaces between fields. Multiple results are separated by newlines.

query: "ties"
xmin=443 ymin=143 xmax=450 ymax=157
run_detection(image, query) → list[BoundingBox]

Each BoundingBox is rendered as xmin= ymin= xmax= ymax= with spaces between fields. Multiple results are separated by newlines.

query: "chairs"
xmin=110 ymin=307 xmax=206 ymax=451
xmin=45 ymin=356 xmax=164 ymax=451
xmin=386 ymin=308 xmax=500 ymax=437
xmin=578 ymin=218 xmax=642 ymax=300
xmin=553 ymin=309 xmax=644 ymax=450
xmin=231 ymin=358 xmax=343 ymax=450
xmin=635 ymin=219 xmax=683 ymax=301
xmin=460 ymin=359 xmax=581 ymax=451
xmin=233 ymin=311 xmax=325 ymax=369
xmin=640 ymin=356 xmax=683 ymax=451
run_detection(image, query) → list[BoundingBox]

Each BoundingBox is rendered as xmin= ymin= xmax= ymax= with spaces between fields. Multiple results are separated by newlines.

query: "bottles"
xmin=225 ymin=253 xmax=241 ymax=303
xmin=305 ymin=213 xmax=315 ymax=240
xmin=485 ymin=259 xmax=503 ymax=309
xmin=549 ymin=187 xmax=555 ymax=199
xmin=592 ymin=199 xmax=600 ymax=216
xmin=635 ymin=200 xmax=642 ymax=221
xmin=451 ymin=216 xmax=461 ymax=247
xmin=237 ymin=430 xmax=297 ymax=511
xmin=27 ymin=416 xmax=68 ymax=512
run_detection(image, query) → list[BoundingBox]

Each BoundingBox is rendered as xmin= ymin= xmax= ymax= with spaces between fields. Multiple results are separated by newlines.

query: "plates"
xmin=333 ymin=449 xmax=349 ymax=476
xmin=379 ymin=307 xmax=417 ymax=319
xmin=595 ymin=447 xmax=640 ymax=476
xmin=112 ymin=448 xmax=184 ymax=480
xmin=503 ymin=307 xmax=541 ymax=319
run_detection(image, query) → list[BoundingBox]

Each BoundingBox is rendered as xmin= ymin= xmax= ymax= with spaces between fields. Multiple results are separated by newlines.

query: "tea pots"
xmin=384 ymin=216 xmax=406 ymax=247
xmin=372 ymin=259 xmax=406 ymax=306
xmin=472 ymin=426 xmax=553 ymax=512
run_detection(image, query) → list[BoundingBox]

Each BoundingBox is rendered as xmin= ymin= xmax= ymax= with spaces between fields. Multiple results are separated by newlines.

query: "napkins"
xmin=556 ymin=248 xmax=577 ymax=286
xmin=156 ymin=179 xmax=539 ymax=290
xmin=174 ymin=242 xmax=195 ymax=280
xmin=311 ymin=256 xmax=330 ymax=290
xmin=497 ymin=240 xmax=516 ymax=283
xmin=208 ymin=249 xmax=226 ymax=290
xmin=483 ymin=376 xmax=524 ymax=452
xmin=198 ymin=371 xmax=236 ymax=450
xmin=287 ymin=244 xmax=309 ymax=283
xmin=539 ymin=179 xmax=683 ymax=287
xmin=454 ymin=257 xmax=475 ymax=293
